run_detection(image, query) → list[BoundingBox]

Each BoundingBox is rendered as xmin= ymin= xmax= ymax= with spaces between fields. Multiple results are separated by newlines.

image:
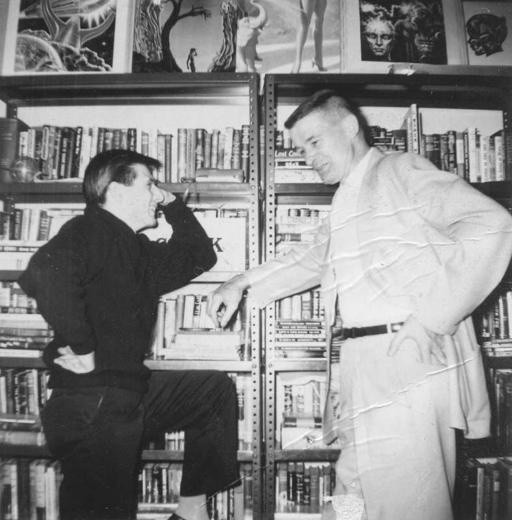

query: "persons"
xmin=186 ymin=47 xmax=198 ymax=72
xmin=362 ymin=8 xmax=397 ymax=58
xmin=19 ymin=148 xmax=241 ymax=519
xmin=463 ymin=14 xmax=508 ymax=57
xmin=289 ymin=0 xmax=329 ymax=75
xmin=206 ymin=88 xmax=510 ymax=518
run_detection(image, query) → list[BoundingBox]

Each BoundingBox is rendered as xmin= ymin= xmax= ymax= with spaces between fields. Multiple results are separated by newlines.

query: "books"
xmin=0 ymin=199 xmax=89 ymax=350
xmin=2 ymin=365 xmax=66 ymax=519
xmin=137 ymin=371 xmax=256 ymax=520
xmin=366 ymin=104 xmax=506 ymax=185
xmin=275 ymin=128 xmax=331 ymax=360
xmin=273 ymin=370 xmax=348 ymax=518
xmin=2 ymin=116 xmax=251 ymax=185
xmin=452 ymin=270 xmax=511 ymax=519
xmin=140 ymin=206 xmax=248 ymax=363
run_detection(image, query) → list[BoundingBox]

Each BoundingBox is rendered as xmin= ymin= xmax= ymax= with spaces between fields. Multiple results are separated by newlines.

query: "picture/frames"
xmin=336 ymin=0 xmax=511 ymax=71
xmin=0 ymin=1 xmax=135 ymax=73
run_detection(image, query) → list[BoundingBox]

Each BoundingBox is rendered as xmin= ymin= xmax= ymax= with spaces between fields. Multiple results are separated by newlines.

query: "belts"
xmin=332 ymin=322 xmax=403 ymax=340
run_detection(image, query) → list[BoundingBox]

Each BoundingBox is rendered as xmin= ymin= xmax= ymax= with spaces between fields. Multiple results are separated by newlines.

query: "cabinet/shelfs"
xmin=0 ymin=72 xmax=261 ymax=519
xmin=262 ymin=72 xmax=511 ymax=518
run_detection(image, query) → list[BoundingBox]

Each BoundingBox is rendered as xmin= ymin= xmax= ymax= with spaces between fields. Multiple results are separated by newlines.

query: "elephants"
xmin=236 ymin=0 xmax=268 ymax=74
